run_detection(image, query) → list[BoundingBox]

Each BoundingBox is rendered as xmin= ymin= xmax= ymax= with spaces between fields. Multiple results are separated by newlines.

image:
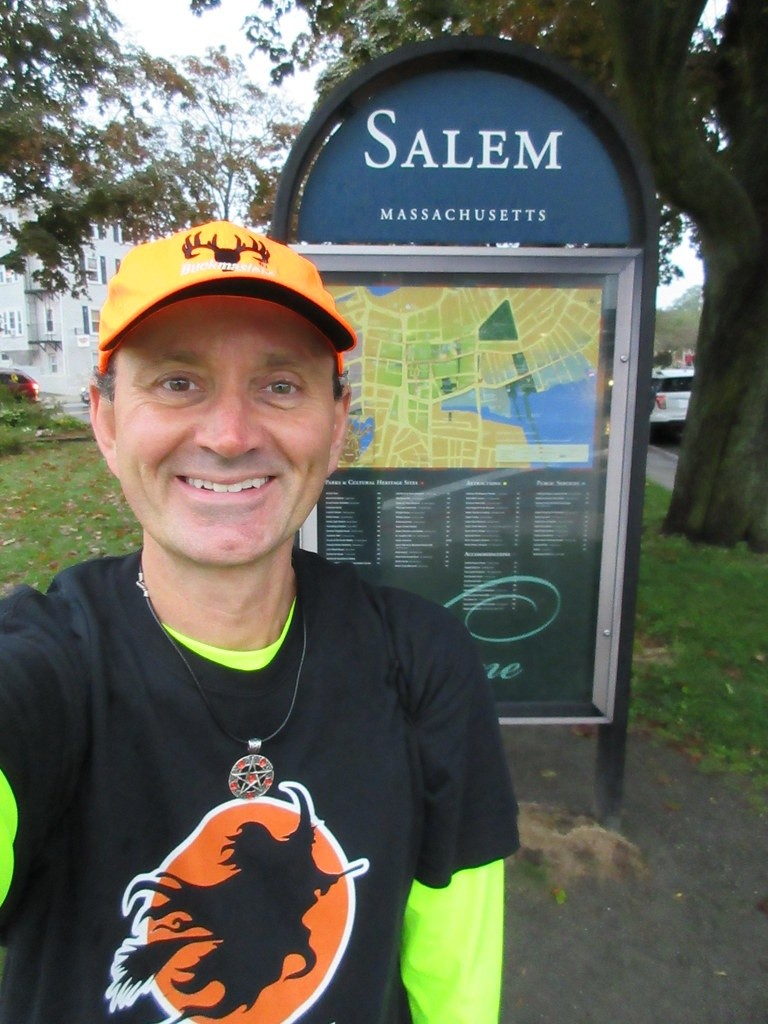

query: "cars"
xmin=0 ymin=368 xmax=43 ymax=405
xmin=80 ymin=386 xmax=91 ymax=405
xmin=650 ymin=368 xmax=695 ymax=439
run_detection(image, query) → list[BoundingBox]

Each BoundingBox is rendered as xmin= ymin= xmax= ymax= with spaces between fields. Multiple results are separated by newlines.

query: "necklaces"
xmin=136 ymin=565 xmax=308 ymax=800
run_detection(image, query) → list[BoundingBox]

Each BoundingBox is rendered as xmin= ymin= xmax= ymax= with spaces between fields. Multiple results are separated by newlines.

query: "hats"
xmin=97 ymin=222 xmax=358 ymax=379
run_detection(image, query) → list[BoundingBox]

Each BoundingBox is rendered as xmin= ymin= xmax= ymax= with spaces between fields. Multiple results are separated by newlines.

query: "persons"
xmin=0 ymin=223 xmax=520 ymax=1023
